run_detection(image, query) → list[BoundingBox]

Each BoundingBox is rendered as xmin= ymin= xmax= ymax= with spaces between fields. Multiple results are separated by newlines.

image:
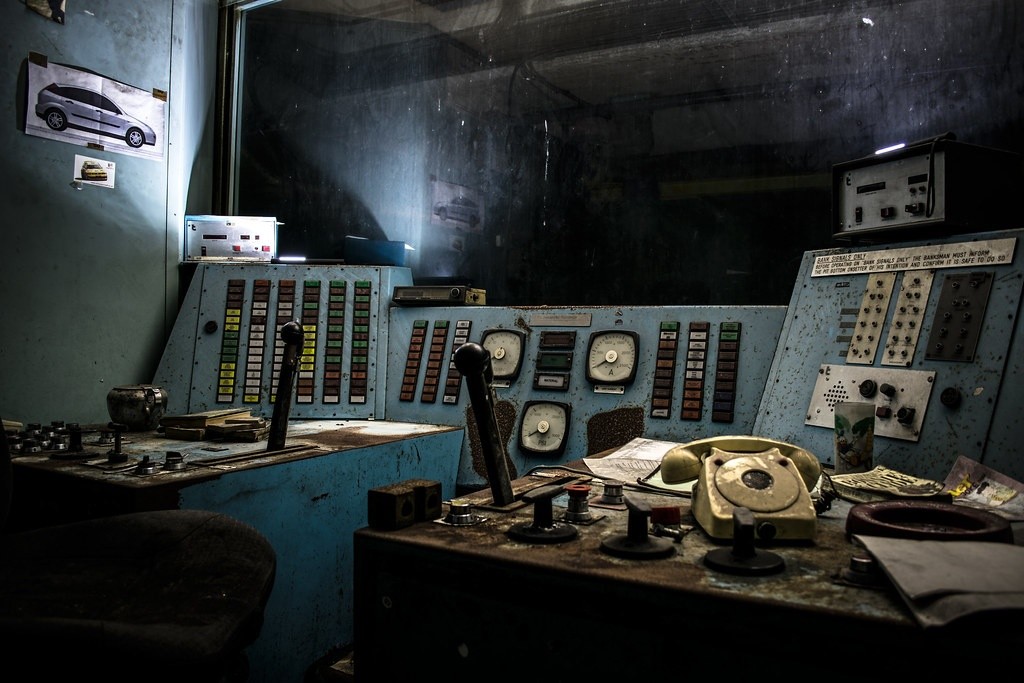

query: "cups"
xmin=833 ymin=402 xmax=876 ymax=474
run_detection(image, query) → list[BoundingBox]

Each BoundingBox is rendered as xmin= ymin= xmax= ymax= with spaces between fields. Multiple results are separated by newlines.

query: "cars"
xmin=34 ymin=82 xmax=156 ymax=148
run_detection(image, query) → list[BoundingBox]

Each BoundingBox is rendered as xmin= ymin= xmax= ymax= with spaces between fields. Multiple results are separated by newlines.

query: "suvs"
xmin=82 ymin=162 xmax=107 ymax=181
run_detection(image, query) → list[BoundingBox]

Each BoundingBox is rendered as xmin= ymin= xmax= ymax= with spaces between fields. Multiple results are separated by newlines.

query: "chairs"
xmin=0 ymin=421 xmax=280 ymax=683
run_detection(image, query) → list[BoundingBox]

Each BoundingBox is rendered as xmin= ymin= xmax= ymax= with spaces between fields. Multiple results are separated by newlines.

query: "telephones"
xmin=661 ymin=433 xmax=821 ymax=547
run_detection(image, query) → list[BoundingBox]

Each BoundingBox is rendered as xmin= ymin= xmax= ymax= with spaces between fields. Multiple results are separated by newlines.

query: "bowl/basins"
xmin=106 ymin=385 xmax=169 ymax=432
